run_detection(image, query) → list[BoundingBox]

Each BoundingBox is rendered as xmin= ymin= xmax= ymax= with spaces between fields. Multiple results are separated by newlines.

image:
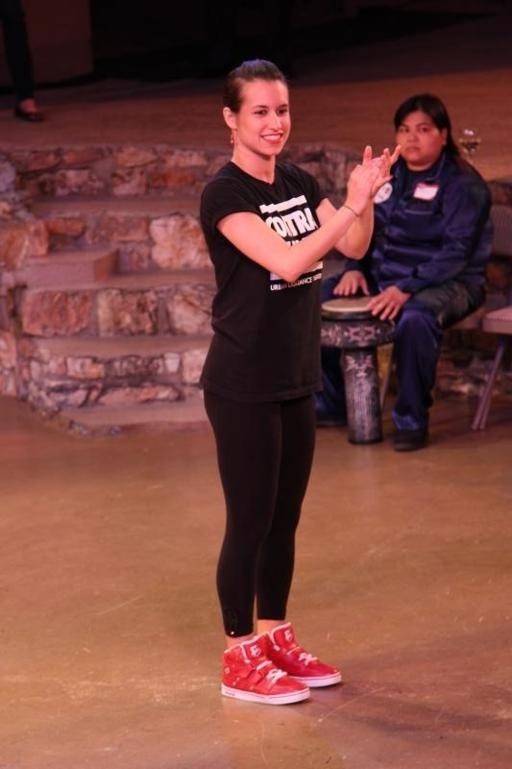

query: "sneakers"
xmin=263 ymin=622 xmax=344 ymax=689
xmin=219 ymin=636 xmax=311 ymax=706
xmin=314 ymin=407 xmax=346 ymax=429
xmin=392 ymin=426 xmax=430 ymax=452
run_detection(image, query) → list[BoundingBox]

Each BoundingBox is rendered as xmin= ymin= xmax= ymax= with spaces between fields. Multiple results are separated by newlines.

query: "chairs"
xmin=377 ymin=203 xmax=512 ymax=414
xmin=470 ymin=305 xmax=512 ymax=432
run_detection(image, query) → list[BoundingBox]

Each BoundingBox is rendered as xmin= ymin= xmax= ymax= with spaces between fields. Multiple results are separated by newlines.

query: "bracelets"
xmin=342 ymin=204 xmax=359 ymax=218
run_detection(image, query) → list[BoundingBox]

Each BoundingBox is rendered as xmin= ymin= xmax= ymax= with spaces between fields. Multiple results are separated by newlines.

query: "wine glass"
xmin=459 ymin=127 xmax=481 ymax=165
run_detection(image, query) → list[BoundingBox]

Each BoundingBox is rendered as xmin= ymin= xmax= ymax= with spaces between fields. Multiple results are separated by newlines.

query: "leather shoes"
xmin=13 ymin=103 xmax=48 ymax=123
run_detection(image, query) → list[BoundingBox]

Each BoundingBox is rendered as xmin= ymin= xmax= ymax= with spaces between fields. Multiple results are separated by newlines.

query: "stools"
xmin=318 ymin=294 xmax=397 ymax=444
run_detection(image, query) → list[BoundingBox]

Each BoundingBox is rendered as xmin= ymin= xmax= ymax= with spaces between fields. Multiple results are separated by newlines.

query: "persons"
xmin=1 ymin=0 xmax=44 ymax=123
xmin=315 ymin=92 xmax=494 ymax=452
xmin=198 ymin=57 xmax=404 ymax=707
